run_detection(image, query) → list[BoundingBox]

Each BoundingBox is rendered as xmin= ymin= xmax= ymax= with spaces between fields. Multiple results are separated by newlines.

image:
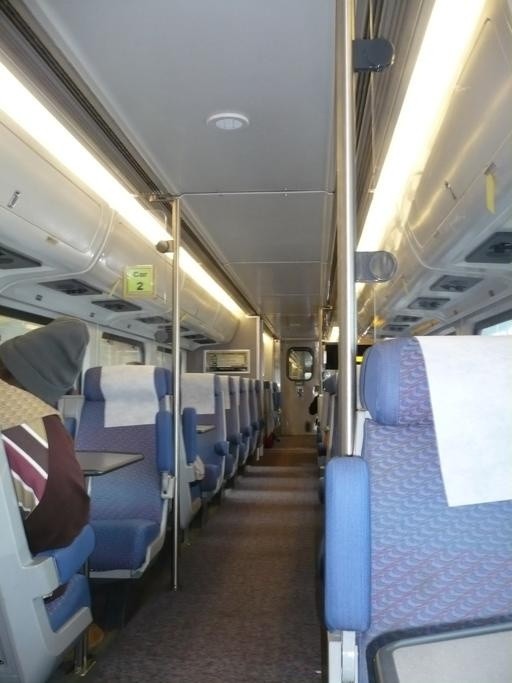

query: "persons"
xmin=0 ymin=319 xmax=106 ymax=661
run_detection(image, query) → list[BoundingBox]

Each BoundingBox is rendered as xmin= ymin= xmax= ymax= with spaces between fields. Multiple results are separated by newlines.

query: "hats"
xmin=0 ymin=317 xmax=89 ymax=406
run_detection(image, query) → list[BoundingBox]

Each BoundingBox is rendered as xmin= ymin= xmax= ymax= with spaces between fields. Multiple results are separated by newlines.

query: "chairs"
xmin=0 ymin=317 xmax=281 ymax=681
xmin=308 ymin=333 xmax=512 ymax=683
